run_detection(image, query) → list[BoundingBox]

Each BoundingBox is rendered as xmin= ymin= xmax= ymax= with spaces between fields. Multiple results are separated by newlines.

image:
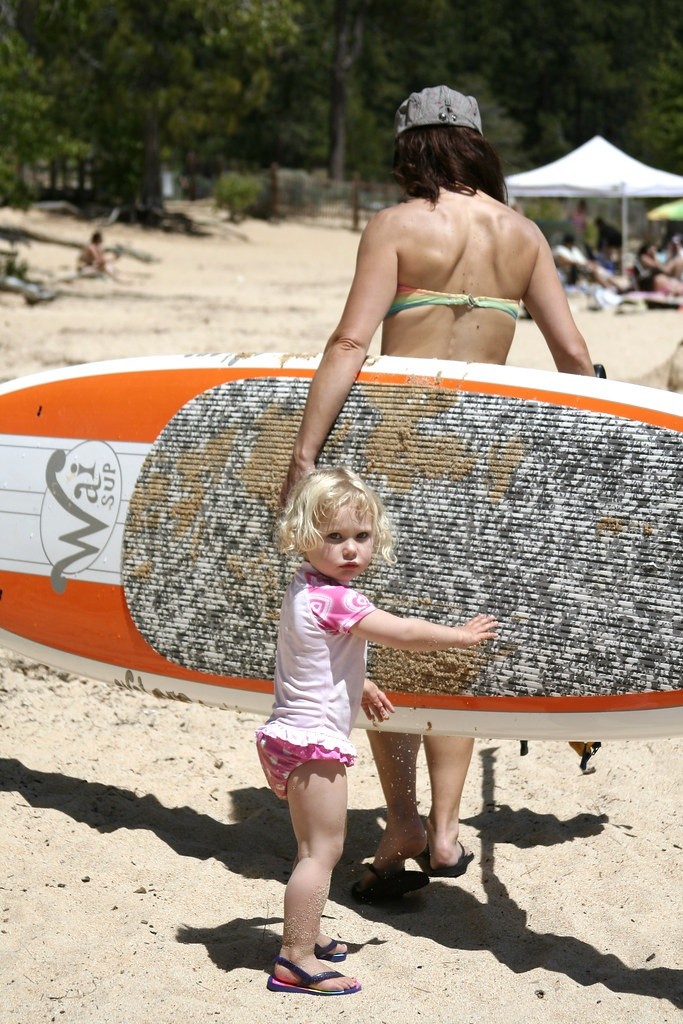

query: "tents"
xmin=504 ymin=135 xmax=683 ymax=276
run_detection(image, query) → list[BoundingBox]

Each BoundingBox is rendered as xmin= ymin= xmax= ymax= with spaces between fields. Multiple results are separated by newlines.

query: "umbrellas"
xmin=645 ymin=199 xmax=683 ymax=221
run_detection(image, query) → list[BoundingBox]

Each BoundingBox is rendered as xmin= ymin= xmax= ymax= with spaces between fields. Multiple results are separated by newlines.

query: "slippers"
xmin=394 ymin=83 xmax=483 ymax=138
xmin=344 ymin=868 xmax=430 ymax=910
xmin=425 ymin=840 xmax=477 ymax=879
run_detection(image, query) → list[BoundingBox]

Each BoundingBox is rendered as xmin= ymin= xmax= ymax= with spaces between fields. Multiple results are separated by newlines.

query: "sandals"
xmin=313 ymin=937 xmax=354 ymax=964
xmin=269 ymin=954 xmax=363 ymax=996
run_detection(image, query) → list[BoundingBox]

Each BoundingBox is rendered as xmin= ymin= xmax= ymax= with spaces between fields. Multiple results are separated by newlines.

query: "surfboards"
xmin=0 ymin=348 xmax=683 ymax=745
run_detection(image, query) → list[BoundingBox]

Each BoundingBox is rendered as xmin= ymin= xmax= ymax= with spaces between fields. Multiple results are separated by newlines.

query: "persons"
xmin=552 ymin=197 xmax=683 ymax=294
xmin=281 ymin=84 xmax=607 ymax=908
xmin=257 ymin=466 xmax=497 ymax=996
xmin=79 ymin=231 xmax=105 ymax=272
